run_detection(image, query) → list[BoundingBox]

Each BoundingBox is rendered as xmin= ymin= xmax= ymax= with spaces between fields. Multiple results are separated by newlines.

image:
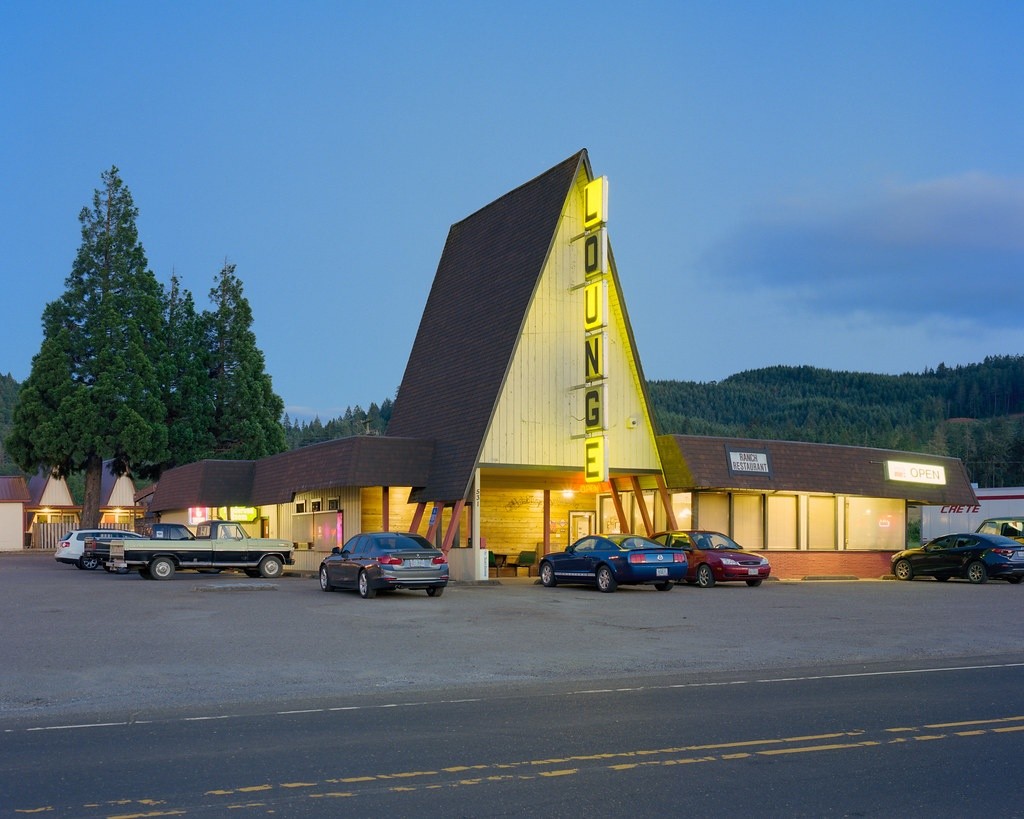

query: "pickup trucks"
xmin=80 ymin=520 xmax=295 ymax=579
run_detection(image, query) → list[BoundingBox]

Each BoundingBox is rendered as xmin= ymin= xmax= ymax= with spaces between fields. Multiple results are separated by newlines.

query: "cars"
xmin=537 ymin=533 xmax=688 ymax=594
xmin=890 ymin=532 xmax=1024 ymax=584
xmin=319 ymin=531 xmax=450 ymax=599
xmin=648 ymin=529 xmax=771 ymax=589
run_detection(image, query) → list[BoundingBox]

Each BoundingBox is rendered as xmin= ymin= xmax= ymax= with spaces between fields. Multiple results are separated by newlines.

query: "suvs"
xmin=973 ymin=517 xmax=1024 ymax=546
xmin=54 ymin=529 xmax=141 ymax=571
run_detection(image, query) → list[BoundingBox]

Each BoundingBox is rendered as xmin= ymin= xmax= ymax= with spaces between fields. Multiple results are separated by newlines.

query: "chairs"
xmin=487 ymin=551 xmax=503 ymax=578
xmin=674 ymin=541 xmax=685 ymax=548
xmin=626 ymin=542 xmax=637 ymax=548
xmin=508 ymin=551 xmax=537 ymax=578
xmin=698 ymin=538 xmax=713 ymax=549
xmin=597 ymin=542 xmax=609 ymax=549
